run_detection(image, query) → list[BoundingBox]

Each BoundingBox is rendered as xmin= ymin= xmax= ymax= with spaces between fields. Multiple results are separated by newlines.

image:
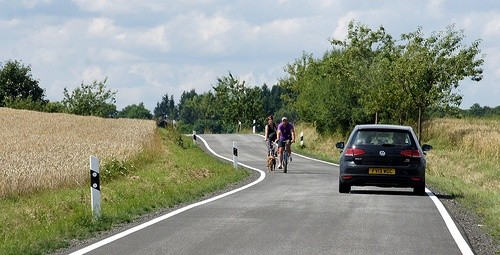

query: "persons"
xmin=392 ymin=132 xmax=405 ymax=144
xmin=358 ymin=133 xmax=372 ymax=145
xmin=277 ymin=117 xmax=294 ymax=169
xmin=265 ymin=116 xmax=277 ymax=158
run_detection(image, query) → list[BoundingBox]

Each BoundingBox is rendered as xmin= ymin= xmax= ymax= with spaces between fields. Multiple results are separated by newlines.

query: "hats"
xmin=282 ymin=117 xmax=287 ymax=121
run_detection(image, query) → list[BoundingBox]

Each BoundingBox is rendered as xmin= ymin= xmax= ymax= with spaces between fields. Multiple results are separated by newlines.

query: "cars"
xmin=336 ymin=124 xmax=434 ymax=197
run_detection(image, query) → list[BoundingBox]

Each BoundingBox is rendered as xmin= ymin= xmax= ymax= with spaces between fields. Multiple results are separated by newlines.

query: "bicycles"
xmin=277 ymin=140 xmax=295 ymax=173
xmin=265 ymin=139 xmax=280 ymax=171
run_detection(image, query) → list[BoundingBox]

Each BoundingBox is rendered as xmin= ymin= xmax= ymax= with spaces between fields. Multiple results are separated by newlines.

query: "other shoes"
xmin=278 ymin=165 xmax=282 ymax=168
xmin=289 ymin=157 xmax=292 ymax=162
